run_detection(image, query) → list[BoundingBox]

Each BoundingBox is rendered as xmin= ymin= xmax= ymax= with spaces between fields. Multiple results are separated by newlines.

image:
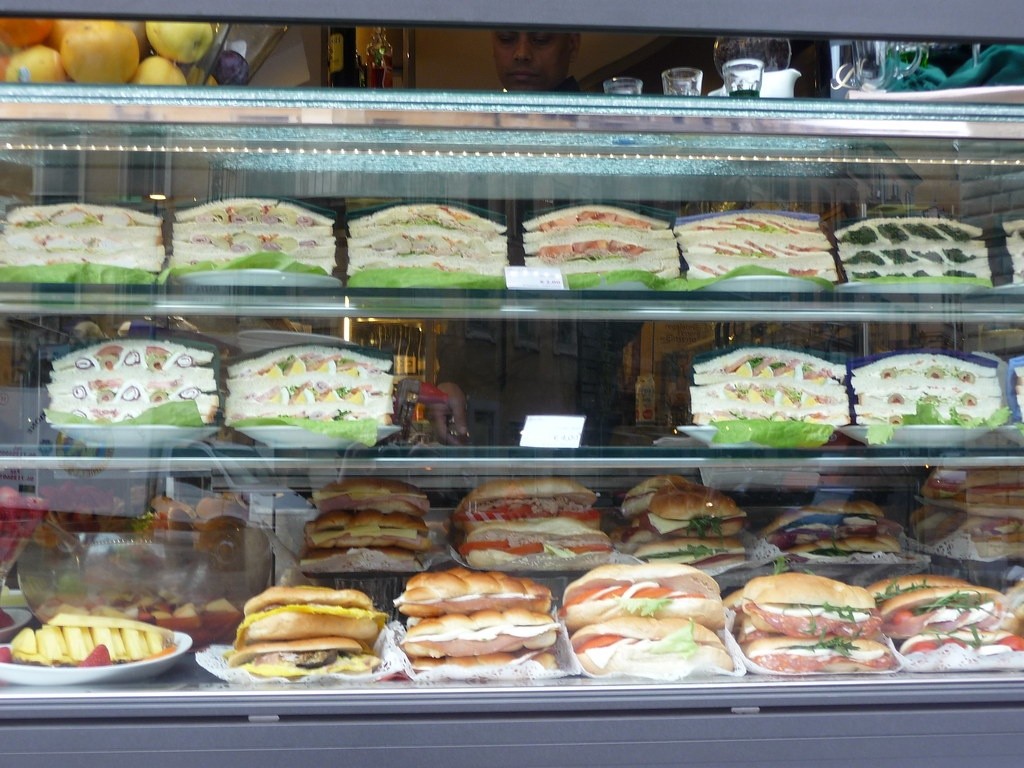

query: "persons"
xmin=424 ymin=27 xmax=643 ymax=445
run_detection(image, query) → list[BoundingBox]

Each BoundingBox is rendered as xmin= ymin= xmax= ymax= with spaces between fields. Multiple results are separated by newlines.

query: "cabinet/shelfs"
xmin=0 ymin=0 xmax=1023 ymax=767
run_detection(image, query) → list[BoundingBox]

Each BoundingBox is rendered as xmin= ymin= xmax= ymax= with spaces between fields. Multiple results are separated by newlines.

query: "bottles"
xmin=364 ymin=27 xmax=393 ymax=90
xmin=713 ymin=36 xmax=792 ymax=80
xmin=329 ymin=24 xmax=358 ymax=89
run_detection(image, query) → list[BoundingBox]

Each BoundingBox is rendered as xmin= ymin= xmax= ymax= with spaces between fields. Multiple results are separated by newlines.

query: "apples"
xmin=0 ymin=17 xmax=221 ymax=90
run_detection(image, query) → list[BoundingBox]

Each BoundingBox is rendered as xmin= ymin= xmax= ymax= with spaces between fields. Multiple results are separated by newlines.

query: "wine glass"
xmin=0 ymin=507 xmax=46 ymax=628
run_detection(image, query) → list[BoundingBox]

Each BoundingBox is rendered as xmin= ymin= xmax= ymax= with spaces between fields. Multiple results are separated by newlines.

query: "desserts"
xmin=11 ymin=612 xmax=170 ymax=664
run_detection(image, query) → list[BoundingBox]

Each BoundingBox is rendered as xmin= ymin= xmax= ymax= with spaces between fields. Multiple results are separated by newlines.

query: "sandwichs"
xmin=0 ymin=196 xmax=1024 ymax=436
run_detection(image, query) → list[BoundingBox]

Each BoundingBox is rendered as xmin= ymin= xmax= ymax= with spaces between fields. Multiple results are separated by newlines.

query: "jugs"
xmin=851 ymin=39 xmax=922 ymax=91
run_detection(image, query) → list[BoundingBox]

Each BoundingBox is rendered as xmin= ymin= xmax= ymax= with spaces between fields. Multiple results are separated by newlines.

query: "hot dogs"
xmin=228 ymin=465 xmax=1024 ymax=684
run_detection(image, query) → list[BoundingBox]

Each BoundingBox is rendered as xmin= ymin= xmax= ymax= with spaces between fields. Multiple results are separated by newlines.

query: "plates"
xmin=840 ymin=425 xmax=994 ymax=447
xmin=234 ymin=424 xmax=403 ymax=449
xmin=994 ymin=425 xmax=1024 ymax=448
xmin=582 ymin=276 xmax=651 ymax=290
xmin=834 ymin=282 xmax=990 ymax=293
xmin=0 ymin=608 xmax=33 ymax=644
xmin=703 ymin=276 xmax=825 ymax=292
xmin=49 ymin=422 xmax=220 ymax=447
xmin=676 ymin=426 xmax=772 ymax=449
xmin=175 ymin=268 xmax=342 ymax=287
xmin=990 ymin=284 xmax=1024 ymax=293
xmin=0 ymin=631 xmax=193 ymax=685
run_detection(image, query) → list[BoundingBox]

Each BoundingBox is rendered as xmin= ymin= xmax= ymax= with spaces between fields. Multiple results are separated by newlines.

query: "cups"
xmin=722 ymin=58 xmax=765 ymax=97
xmin=603 ymin=77 xmax=643 ymax=95
xmin=661 ymin=67 xmax=703 ymax=97
